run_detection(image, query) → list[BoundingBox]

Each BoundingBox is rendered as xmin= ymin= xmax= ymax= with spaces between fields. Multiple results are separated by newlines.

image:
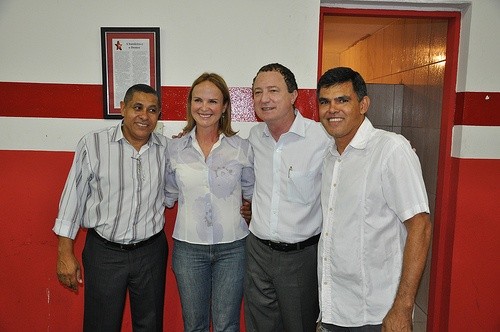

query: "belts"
xmin=256 ymin=237 xmax=318 ymax=253
xmin=90 ymin=228 xmax=163 ymax=249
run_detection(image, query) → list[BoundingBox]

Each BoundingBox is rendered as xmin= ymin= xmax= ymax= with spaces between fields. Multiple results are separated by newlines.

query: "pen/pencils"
xmin=287 ymin=166 xmax=293 ymax=178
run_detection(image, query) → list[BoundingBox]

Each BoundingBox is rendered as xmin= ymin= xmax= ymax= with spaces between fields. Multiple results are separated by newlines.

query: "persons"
xmin=172 ymin=64 xmax=335 ymax=332
xmin=315 ymin=66 xmax=432 ymax=332
xmin=52 ymin=83 xmax=252 ymax=332
xmin=162 ymin=73 xmax=255 ymax=331
